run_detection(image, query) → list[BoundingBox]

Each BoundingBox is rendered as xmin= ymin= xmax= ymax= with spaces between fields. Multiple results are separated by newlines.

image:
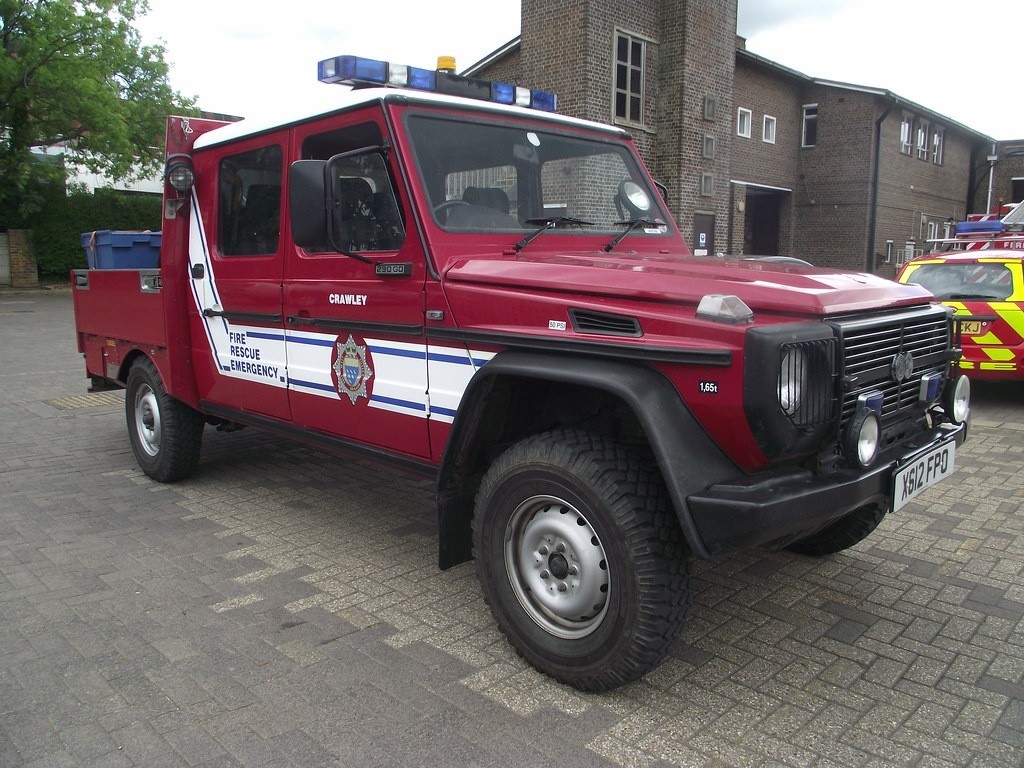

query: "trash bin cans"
xmin=80 ymin=230 xmax=162 ymax=270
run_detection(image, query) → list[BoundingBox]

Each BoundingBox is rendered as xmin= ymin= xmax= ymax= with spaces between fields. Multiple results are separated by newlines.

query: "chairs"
xmin=244 ymin=178 xmax=510 ymax=253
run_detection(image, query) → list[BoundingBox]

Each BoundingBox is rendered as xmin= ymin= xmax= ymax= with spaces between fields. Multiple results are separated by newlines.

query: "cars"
xmin=893 ymin=221 xmax=1024 ymax=381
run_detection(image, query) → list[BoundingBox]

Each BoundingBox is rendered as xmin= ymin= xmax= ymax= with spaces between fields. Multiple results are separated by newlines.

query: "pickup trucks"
xmin=70 ymin=56 xmax=970 ymax=694
